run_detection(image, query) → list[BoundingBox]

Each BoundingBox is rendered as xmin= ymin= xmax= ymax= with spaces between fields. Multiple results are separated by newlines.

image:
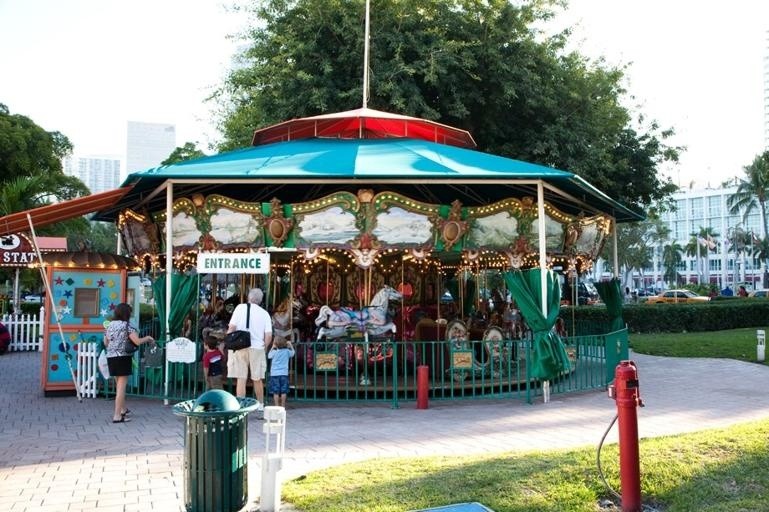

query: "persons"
xmin=217 ymin=286 xmax=274 ymax=422
xmin=737 ymin=285 xmax=749 ymax=297
xmin=202 ymin=335 xmax=227 ymax=393
xmin=101 ymin=302 xmax=155 ymax=425
xmin=556 ymin=316 xmax=566 ymax=337
xmin=708 ymin=287 xmax=719 ymax=300
xmin=622 ymin=286 xmax=631 ymax=304
xmin=267 ymin=334 xmax=296 ymax=409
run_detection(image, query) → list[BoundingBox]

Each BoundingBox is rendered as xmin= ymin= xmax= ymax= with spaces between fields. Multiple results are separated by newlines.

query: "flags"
xmin=723 ymin=232 xmax=734 ymax=254
xmin=696 ymin=236 xmax=710 ymax=247
xmin=752 ymin=230 xmax=761 ymax=246
xmin=706 ymin=234 xmax=722 ymax=249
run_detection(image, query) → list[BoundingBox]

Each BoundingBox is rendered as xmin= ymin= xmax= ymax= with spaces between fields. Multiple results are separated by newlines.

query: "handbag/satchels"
xmin=224 ymin=331 xmax=251 ymax=350
xmin=125 ymin=322 xmax=139 ymax=353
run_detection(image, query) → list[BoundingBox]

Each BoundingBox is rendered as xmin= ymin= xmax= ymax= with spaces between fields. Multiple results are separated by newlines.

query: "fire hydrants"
xmin=607 ymin=359 xmax=645 ymax=511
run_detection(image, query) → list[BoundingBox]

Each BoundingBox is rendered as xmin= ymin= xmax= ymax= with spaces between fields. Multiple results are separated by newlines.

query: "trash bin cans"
xmin=756 ymin=330 xmax=766 ymax=362
xmin=172 ymin=389 xmax=259 ymax=512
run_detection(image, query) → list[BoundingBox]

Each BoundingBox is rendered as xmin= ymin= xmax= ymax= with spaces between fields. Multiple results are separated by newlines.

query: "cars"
xmin=645 ymin=290 xmax=711 ymax=303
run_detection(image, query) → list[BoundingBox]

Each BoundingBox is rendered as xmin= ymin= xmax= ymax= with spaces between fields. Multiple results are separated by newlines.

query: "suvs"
xmin=561 ymin=283 xmax=598 ymax=305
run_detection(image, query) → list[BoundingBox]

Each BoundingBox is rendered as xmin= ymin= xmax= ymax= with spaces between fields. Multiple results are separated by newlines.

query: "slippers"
xmin=112 ymin=408 xmax=132 ymax=423
